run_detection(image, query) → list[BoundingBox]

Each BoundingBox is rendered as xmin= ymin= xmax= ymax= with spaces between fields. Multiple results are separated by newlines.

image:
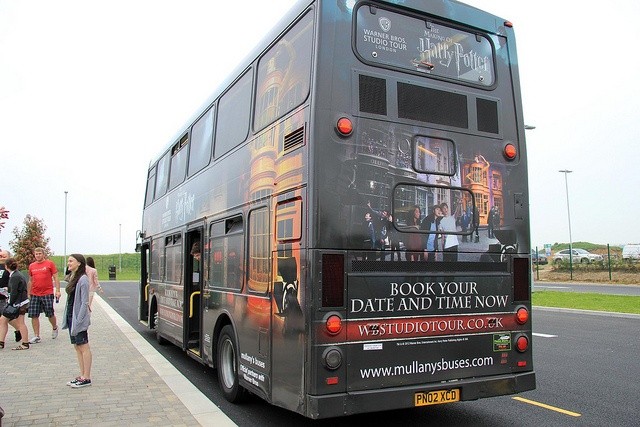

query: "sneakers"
xmin=14 ymin=330 xmax=22 ymax=342
xmin=71 ymin=379 xmax=92 ymax=388
xmin=66 ymin=377 xmax=83 ymax=386
xmin=12 ymin=342 xmax=29 ymax=350
xmin=28 ymin=336 xmax=41 ymax=344
xmin=52 ymin=326 xmax=58 ymax=339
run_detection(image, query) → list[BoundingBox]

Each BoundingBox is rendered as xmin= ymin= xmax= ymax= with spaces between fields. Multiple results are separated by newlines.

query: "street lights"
xmin=558 ymin=169 xmax=572 ymax=265
xmin=64 ymin=190 xmax=68 ymax=275
xmin=119 ymin=223 xmax=122 ymax=270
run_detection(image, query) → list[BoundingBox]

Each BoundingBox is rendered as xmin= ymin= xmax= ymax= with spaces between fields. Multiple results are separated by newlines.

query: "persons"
xmin=0 ymin=250 xmax=22 ymax=342
xmin=27 ymin=247 xmax=60 ymax=344
xmin=420 ymin=204 xmax=443 ymax=261
xmin=461 ymin=205 xmax=479 ymax=244
xmin=61 ymin=253 xmax=92 ymax=389
xmin=376 ymin=209 xmax=389 ymax=260
xmin=487 ymin=203 xmax=501 ymax=238
xmin=437 ymin=198 xmax=464 ymax=262
xmin=85 ymin=255 xmax=105 ymax=312
xmin=405 ymin=204 xmax=423 ymax=261
xmin=0 ymin=258 xmax=30 ymax=350
xmin=191 ymin=242 xmax=200 ymax=262
xmin=364 ymin=212 xmax=378 ymax=260
xmin=387 ymin=213 xmax=402 ymax=261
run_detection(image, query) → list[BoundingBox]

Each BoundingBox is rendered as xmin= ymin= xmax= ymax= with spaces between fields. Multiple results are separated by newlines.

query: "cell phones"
xmin=56 ymin=296 xmax=59 ymax=303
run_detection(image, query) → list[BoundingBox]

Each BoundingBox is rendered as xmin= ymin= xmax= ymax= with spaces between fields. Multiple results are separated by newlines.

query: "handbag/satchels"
xmin=3 ymin=273 xmax=25 ymax=319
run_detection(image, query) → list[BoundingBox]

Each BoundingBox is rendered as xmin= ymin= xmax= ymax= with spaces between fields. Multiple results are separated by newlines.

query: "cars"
xmin=621 ymin=244 xmax=640 ymax=260
xmin=531 ymin=249 xmax=547 ymax=263
xmin=552 ymin=247 xmax=603 ymax=264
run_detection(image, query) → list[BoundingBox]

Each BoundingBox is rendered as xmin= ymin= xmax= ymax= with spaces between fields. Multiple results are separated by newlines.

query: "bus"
xmin=135 ymin=0 xmax=535 ymax=418
